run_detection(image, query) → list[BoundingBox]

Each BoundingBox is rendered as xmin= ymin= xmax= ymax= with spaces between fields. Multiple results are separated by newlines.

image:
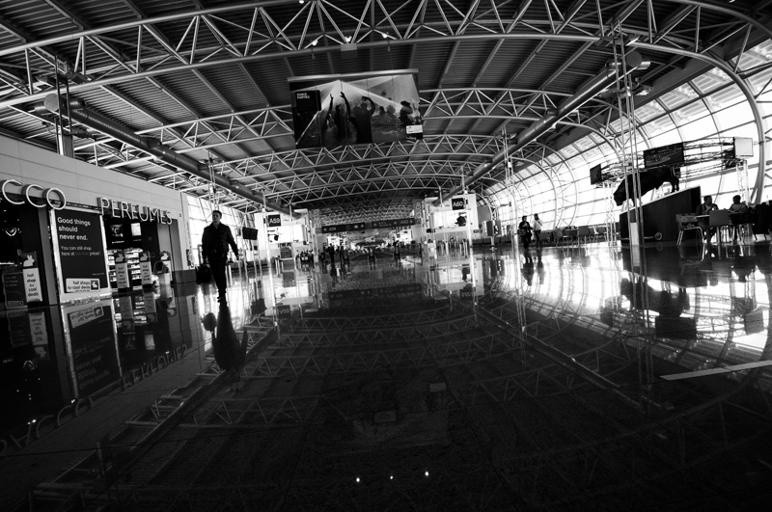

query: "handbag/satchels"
xmin=196 ymin=263 xmax=214 ymax=284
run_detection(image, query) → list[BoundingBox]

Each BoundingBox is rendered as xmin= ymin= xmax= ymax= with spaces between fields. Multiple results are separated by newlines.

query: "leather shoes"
xmin=218 ymin=295 xmax=225 ymax=301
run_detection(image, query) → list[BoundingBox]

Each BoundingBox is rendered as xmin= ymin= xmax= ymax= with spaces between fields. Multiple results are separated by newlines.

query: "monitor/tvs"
xmin=598 ymin=307 xmax=615 ymax=329
xmin=590 ymin=164 xmax=602 ymax=184
xmin=287 ymin=68 xmax=424 ymax=147
xmin=242 ymin=227 xmax=257 ymax=240
xmin=742 ymin=311 xmax=765 ymax=334
xmin=734 ymin=137 xmax=754 ymax=157
xmin=643 ymin=141 xmax=686 ymax=170
xmin=250 ymin=298 xmax=267 ymax=315
xmin=648 ymin=314 xmax=702 ymax=342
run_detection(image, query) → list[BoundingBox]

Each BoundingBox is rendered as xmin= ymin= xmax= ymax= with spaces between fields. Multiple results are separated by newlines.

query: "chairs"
xmin=554 ymin=225 xmax=606 ymax=247
xmin=675 ymin=204 xmax=757 ymax=259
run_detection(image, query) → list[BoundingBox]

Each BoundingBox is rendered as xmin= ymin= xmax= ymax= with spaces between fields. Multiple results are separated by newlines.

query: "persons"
xmin=315 ymin=88 xmax=421 ymax=144
xmin=318 ymin=210 xmax=543 ymax=269
xmin=201 ymin=209 xmax=240 ymax=301
xmin=724 ymin=194 xmax=747 ymax=241
xmin=699 ymin=196 xmax=720 ymax=241
